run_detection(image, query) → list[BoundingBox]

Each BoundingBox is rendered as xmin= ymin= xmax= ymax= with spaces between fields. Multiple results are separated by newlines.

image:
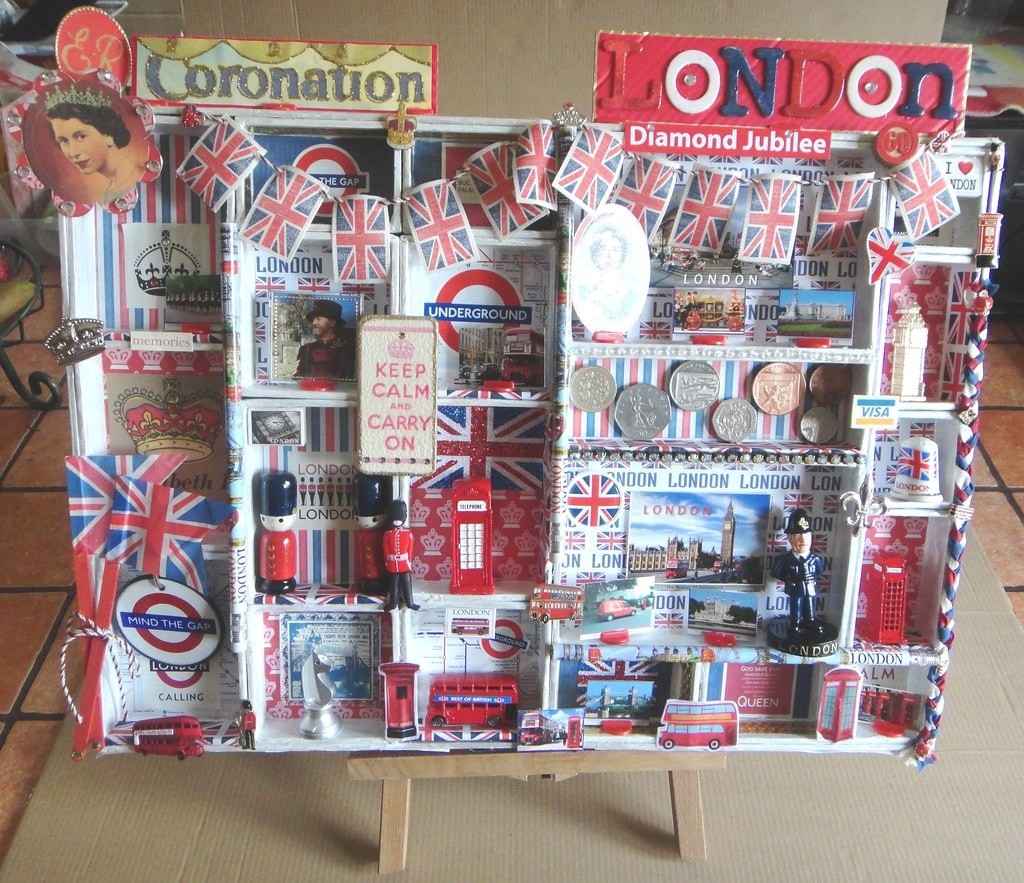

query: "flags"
xmin=166 ymin=108 xmax=961 ymax=285
xmin=405 ymin=402 xmax=547 ymax=497
xmin=869 ymin=415 xmax=952 ymax=502
xmin=556 ymin=455 xmax=848 ymax=625
xmin=63 ymin=449 xmax=237 ymax=597
xmin=943 ymin=267 xmax=984 ymax=404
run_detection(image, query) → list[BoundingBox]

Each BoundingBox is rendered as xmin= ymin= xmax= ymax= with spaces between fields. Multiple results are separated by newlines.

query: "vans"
xmin=598 ymin=599 xmax=638 ymax=622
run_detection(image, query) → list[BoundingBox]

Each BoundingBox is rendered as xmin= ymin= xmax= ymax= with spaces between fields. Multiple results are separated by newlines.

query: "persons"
xmin=577 ymin=224 xmax=637 ymax=327
xmin=47 ymin=84 xmax=153 ymax=210
xmin=380 ymin=498 xmax=423 ymax=613
xmin=771 ymin=510 xmax=828 ymax=637
xmin=289 ymin=297 xmax=358 ymax=384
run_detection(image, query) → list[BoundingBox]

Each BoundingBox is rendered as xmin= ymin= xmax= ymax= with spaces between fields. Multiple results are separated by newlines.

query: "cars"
xmin=459 ymin=360 xmax=501 ymax=386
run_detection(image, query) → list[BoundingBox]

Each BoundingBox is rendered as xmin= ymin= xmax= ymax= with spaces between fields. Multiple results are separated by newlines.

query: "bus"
xmin=520 ymin=712 xmax=555 ymax=745
xmin=426 ymin=673 xmax=518 ymax=729
xmin=713 ymin=561 xmax=727 ymax=573
xmin=504 ymin=328 xmax=545 ymax=386
xmin=665 ymin=558 xmax=688 ymax=579
xmin=133 ymin=716 xmax=206 ymax=761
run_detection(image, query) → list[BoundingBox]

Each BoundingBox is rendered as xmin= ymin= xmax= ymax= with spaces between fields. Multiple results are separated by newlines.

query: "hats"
xmin=307 ymin=299 xmax=345 ymax=328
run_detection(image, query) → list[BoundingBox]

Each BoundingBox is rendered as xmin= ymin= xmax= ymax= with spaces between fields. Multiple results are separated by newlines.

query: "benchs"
xmin=344 ymin=747 xmax=729 ymax=875
